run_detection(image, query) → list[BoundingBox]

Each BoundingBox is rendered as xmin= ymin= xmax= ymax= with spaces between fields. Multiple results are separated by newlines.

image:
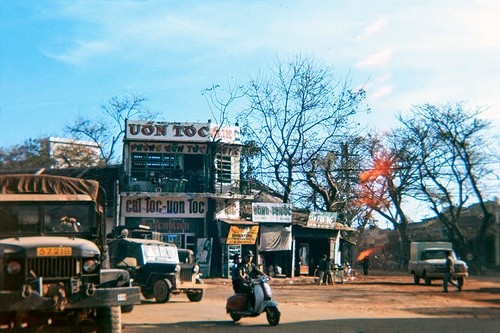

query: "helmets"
xmin=243 ymin=250 xmax=254 ymax=257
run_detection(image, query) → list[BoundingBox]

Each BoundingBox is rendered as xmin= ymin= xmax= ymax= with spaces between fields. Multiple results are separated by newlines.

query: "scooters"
xmin=226 ymin=275 xmax=280 ymax=326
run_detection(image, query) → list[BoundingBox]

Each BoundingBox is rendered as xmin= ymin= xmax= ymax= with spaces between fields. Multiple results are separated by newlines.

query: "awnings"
xmin=217 ymin=218 xmax=258 ymax=225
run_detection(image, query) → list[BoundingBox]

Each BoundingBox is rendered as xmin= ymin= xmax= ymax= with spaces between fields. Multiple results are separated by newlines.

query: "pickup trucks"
xmin=408 ymin=247 xmax=469 ymax=287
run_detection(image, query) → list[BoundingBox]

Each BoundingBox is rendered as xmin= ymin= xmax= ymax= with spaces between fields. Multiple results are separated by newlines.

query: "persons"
xmin=317 ymin=255 xmax=334 ymax=284
xmin=441 ymin=250 xmax=453 ymax=293
xmin=362 ymin=255 xmax=369 ymax=276
xmin=60 ymin=215 xmax=79 ymax=232
xmin=231 ymin=250 xmax=267 ymax=308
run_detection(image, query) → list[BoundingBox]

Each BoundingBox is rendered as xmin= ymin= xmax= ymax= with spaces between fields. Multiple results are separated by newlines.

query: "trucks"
xmin=0 ymin=174 xmax=142 ymax=333
xmin=107 ymin=235 xmax=209 ymax=303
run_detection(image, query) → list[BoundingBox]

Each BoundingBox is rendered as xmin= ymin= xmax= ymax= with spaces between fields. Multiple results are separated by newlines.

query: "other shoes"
xmin=458 ymin=287 xmax=461 ymax=291
xmin=333 ymin=283 xmax=335 ymax=284
xmin=442 ymin=290 xmax=448 ymax=292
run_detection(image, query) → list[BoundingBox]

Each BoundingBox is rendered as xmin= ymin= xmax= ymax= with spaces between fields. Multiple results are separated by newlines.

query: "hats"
xmin=234 ymin=254 xmax=241 ymax=259
